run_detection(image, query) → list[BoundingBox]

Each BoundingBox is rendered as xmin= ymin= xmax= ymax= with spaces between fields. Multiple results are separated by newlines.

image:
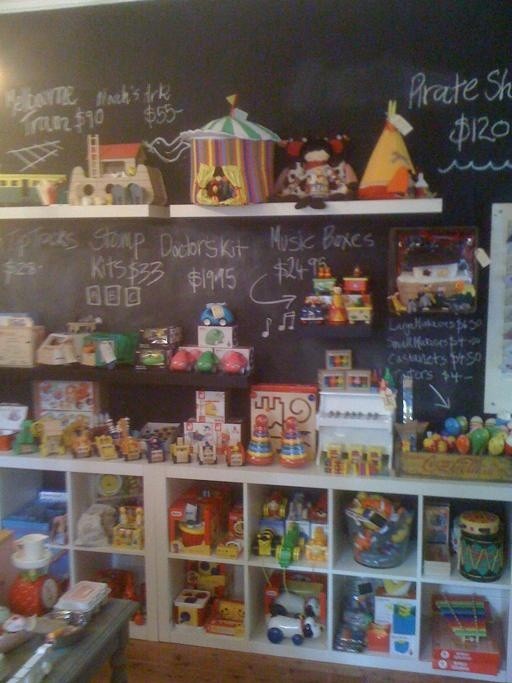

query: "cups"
xmin=1 ymin=429 xmax=15 ymax=450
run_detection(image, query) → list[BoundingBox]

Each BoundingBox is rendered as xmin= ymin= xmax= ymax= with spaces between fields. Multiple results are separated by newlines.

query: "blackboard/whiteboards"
xmin=0 ymin=0 xmax=512 ymax=453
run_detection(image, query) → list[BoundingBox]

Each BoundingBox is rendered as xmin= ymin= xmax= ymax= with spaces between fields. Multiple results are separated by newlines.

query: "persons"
xmin=457 ymin=278 xmax=476 ymax=310
xmin=419 ymin=286 xmax=436 ymax=310
xmin=288 ymin=139 xmax=343 ymax=209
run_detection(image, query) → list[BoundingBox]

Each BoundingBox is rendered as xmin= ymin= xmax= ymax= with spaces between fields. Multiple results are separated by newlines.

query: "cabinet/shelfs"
xmin=0 ymin=198 xmax=444 ymax=391
xmin=0 ymin=453 xmax=159 ymax=643
xmin=156 ymin=459 xmax=512 ymax=683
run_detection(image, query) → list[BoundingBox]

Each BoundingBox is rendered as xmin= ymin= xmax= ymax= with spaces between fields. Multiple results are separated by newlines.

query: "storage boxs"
xmin=401 ymin=451 xmax=512 ymax=483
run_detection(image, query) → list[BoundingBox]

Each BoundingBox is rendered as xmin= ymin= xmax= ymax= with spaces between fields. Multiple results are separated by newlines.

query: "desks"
xmin=0 ymin=598 xmax=141 ymax=683
